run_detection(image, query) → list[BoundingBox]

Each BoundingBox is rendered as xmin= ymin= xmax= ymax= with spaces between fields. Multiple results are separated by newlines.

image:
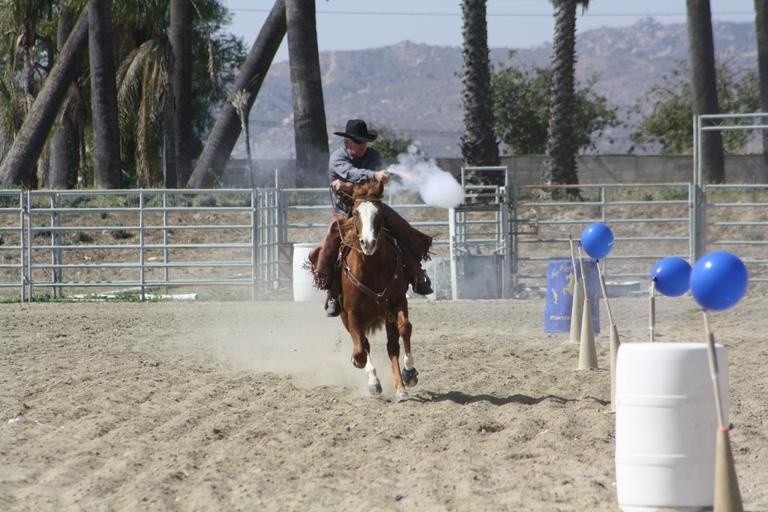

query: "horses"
xmin=328 ymin=178 xmax=419 ymax=404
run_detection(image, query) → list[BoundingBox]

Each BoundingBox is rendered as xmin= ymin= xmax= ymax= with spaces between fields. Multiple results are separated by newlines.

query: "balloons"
xmin=580 ymin=223 xmax=614 ymax=264
xmin=687 ymin=249 xmax=749 ymax=313
xmin=649 ymin=256 xmax=693 ymax=298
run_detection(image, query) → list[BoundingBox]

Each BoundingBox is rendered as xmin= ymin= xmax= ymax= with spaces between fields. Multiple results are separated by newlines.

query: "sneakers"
xmin=413 ymin=281 xmax=433 ymax=295
xmin=326 ymin=297 xmax=343 ymax=317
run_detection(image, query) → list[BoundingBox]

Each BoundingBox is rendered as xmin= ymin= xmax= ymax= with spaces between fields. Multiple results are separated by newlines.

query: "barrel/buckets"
xmin=545 ymin=255 xmax=601 ymax=335
xmin=290 ymin=242 xmax=328 ymax=302
xmin=615 ymin=340 xmax=727 ymax=511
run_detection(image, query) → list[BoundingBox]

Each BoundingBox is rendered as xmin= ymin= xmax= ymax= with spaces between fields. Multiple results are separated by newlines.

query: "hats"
xmin=333 ymin=119 xmax=378 ymax=142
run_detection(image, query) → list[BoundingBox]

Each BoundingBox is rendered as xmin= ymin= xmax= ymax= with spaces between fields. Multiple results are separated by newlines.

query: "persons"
xmin=315 ymin=119 xmax=434 ymax=318
